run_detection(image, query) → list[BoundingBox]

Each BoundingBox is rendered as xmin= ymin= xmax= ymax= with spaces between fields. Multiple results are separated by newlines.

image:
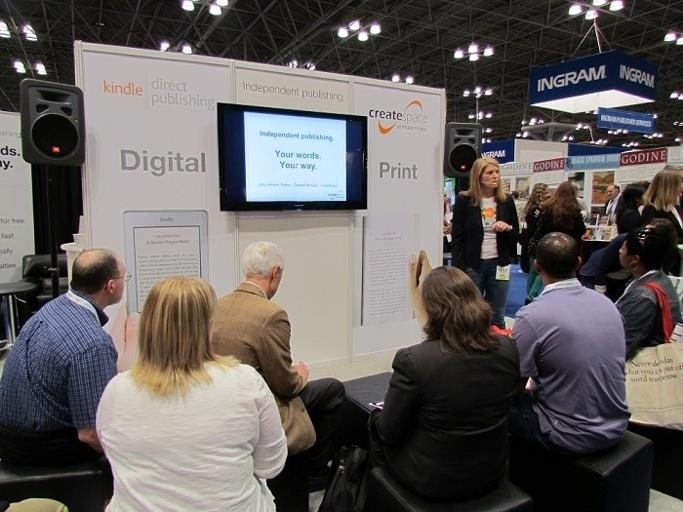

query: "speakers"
xmin=20 ymin=78 xmax=86 ymax=168
xmin=443 ymin=122 xmax=482 ymax=179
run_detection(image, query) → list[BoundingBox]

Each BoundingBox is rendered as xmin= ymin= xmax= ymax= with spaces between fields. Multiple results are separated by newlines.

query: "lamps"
xmin=675 ymin=138 xmax=680 ymax=142
xmin=524 ymin=21 xmax=656 ymax=113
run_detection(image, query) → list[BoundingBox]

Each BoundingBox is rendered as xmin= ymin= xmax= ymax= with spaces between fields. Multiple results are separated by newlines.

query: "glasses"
xmin=102 ymin=268 xmax=132 ymax=291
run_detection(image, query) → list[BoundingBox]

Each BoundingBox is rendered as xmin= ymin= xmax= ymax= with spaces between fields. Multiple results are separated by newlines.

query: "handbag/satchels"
xmin=624 ymin=281 xmax=683 ymax=432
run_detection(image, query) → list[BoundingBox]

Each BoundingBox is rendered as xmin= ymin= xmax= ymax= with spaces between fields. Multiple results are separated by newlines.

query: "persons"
xmin=508 ymin=233 xmax=631 ymax=457
xmin=524 ymin=183 xmax=551 ymax=304
xmin=451 ymin=157 xmax=520 ymax=328
xmin=614 ymin=184 xmax=646 ymax=233
xmin=443 ymin=194 xmax=453 ymax=251
xmin=569 ymin=182 xmax=589 ymax=219
xmin=0 ymin=248 xmax=127 ymax=453
xmin=603 ymin=184 xmax=622 ymax=224
xmin=102 ymin=276 xmax=287 ymax=512
xmin=510 ymin=192 xmax=523 ymax=220
xmin=612 ymin=219 xmax=683 ymax=362
xmin=551 ymin=183 xmax=586 ymax=236
xmin=217 ymin=240 xmax=348 ymax=478
xmin=642 ymin=170 xmax=683 ymax=244
xmin=365 ymin=266 xmax=521 ymax=507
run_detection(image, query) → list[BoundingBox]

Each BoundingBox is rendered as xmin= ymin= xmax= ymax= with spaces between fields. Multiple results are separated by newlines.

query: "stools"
xmin=514 ymin=424 xmax=651 ymax=512
xmin=0 ymin=282 xmax=36 ymax=352
xmin=371 ymin=463 xmax=532 ymax=512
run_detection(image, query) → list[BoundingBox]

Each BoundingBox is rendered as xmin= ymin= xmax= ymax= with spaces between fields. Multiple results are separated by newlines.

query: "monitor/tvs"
xmin=216 ymin=102 xmax=368 ymax=211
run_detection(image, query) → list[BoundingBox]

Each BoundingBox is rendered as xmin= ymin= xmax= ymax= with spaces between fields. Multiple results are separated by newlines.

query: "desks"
xmin=331 ymin=370 xmax=392 ymax=421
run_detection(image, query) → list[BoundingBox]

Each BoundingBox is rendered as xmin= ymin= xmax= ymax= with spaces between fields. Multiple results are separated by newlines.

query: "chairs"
xmin=0 ymin=449 xmax=112 ymax=511
